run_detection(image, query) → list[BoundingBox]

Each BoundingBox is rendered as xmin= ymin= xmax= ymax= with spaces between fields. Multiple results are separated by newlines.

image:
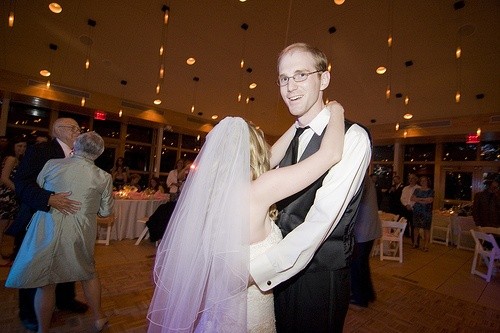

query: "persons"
xmin=109 ymin=158 xmax=126 ymax=189
xmin=5 ymin=117 xmax=89 ymax=332
xmin=370 ymin=173 xmax=410 ymax=238
xmin=410 ymin=175 xmax=434 ymax=252
xmin=471 ymin=172 xmax=500 ymax=261
xmin=136 ymin=177 xmax=165 ymax=193
xmin=241 ymin=43 xmax=372 ymax=333
xmin=401 ymin=174 xmax=420 ymax=245
xmin=145 ymin=101 xmax=344 ymax=333
xmin=166 ymin=157 xmax=193 ymax=199
xmin=349 ymin=176 xmax=382 ymax=308
xmin=6 ymin=131 xmax=109 ymax=333
xmin=0 ymin=131 xmax=48 ymax=267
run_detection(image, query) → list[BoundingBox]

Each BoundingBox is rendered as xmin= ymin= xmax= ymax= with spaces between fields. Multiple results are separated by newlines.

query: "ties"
xmin=271 ymin=125 xmax=310 ymax=214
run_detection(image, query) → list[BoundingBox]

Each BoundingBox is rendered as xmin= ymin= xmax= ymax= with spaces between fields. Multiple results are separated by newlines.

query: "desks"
xmin=97 ymin=196 xmax=169 ymax=240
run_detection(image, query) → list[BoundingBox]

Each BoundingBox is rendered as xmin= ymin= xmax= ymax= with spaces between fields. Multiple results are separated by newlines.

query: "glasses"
xmin=274 ymin=69 xmax=325 ymax=87
xmin=58 ymin=125 xmax=81 ymax=133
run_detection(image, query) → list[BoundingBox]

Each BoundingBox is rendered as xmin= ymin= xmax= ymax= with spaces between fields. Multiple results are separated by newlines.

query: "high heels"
xmin=95 ymin=312 xmax=111 ymax=331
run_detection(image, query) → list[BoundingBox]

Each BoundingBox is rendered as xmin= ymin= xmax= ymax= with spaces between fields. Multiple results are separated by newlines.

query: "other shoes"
xmin=349 ymin=293 xmax=377 ymax=308
xmin=17 ymin=309 xmax=39 ymax=330
xmin=55 ymin=298 xmax=89 ymax=314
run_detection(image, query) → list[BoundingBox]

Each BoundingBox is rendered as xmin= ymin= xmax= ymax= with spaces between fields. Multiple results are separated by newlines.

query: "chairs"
xmin=368 ymin=212 xmax=408 ymax=263
xmin=134 ymin=201 xmax=175 ymax=247
xmin=95 ymin=212 xmax=115 ymax=245
xmin=428 ymin=213 xmax=500 ymax=281
